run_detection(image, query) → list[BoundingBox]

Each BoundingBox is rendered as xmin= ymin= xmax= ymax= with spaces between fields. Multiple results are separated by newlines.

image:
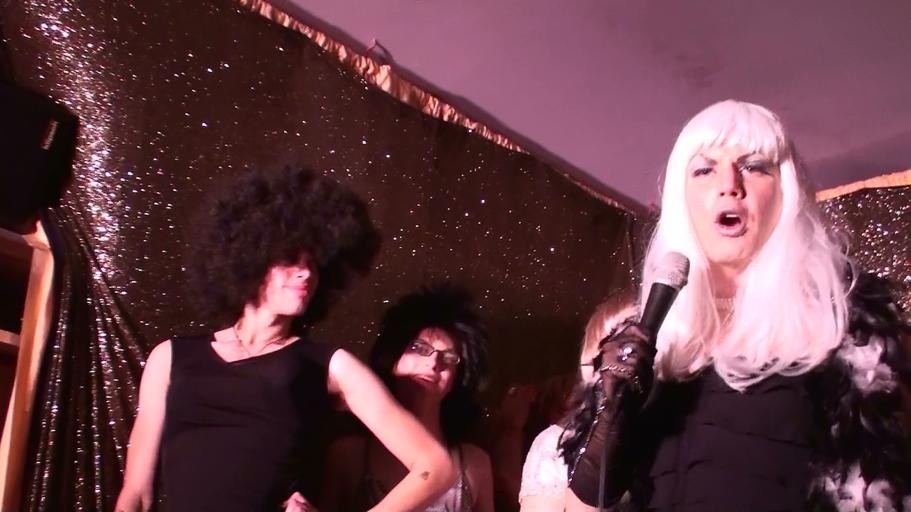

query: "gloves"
xmin=567 ymin=322 xmax=658 ymax=508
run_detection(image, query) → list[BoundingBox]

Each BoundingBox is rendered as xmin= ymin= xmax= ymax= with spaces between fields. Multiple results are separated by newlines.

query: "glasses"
xmin=407 ymin=338 xmax=461 ymax=367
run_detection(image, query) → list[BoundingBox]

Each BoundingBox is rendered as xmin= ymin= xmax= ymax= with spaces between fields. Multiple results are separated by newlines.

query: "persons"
xmin=556 ymin=98 xmax=911 ymax=512
xmin=519 ymin=286 xmax=642 ymax=512
xmin=113 ymin=161 xmax=460 ymax=512
xmin=316 ymin=280 xmax=496 ymax=511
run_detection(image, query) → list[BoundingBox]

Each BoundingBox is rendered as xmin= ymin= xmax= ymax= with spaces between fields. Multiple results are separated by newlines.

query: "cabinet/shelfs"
xmin=0 ymin=217 xmax=55 ymax=511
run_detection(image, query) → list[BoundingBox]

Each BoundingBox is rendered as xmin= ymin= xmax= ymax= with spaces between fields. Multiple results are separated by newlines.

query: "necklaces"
xmin=713 ymin=297 xmax=735 ymax=310
xmin=232 ymin=325 xmax=287 ymax=357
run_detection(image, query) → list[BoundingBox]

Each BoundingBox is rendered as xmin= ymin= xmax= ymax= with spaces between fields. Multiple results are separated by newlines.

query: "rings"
xmin=622 ymin=347 xmax=633 ymax=361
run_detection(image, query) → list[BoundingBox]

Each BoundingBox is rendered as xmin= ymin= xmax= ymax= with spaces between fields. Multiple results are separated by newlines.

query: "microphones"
xmin=614 ymin=249 xmax=688 ymax=390
